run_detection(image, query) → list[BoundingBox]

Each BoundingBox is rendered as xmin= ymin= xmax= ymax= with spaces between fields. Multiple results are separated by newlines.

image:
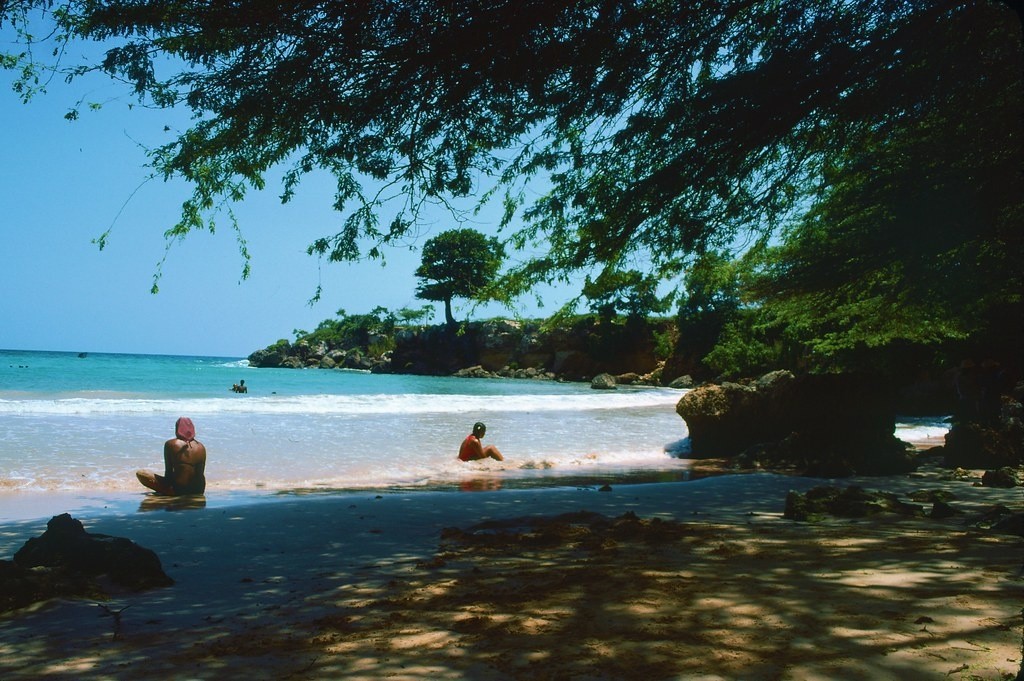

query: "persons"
xmin=459 ymin=422 xmax=504 ymax=462
xmin=229 ymin=380 xmax=247 ymax=393
xmin=136 ymin=417 xmax=206 ymax=495
